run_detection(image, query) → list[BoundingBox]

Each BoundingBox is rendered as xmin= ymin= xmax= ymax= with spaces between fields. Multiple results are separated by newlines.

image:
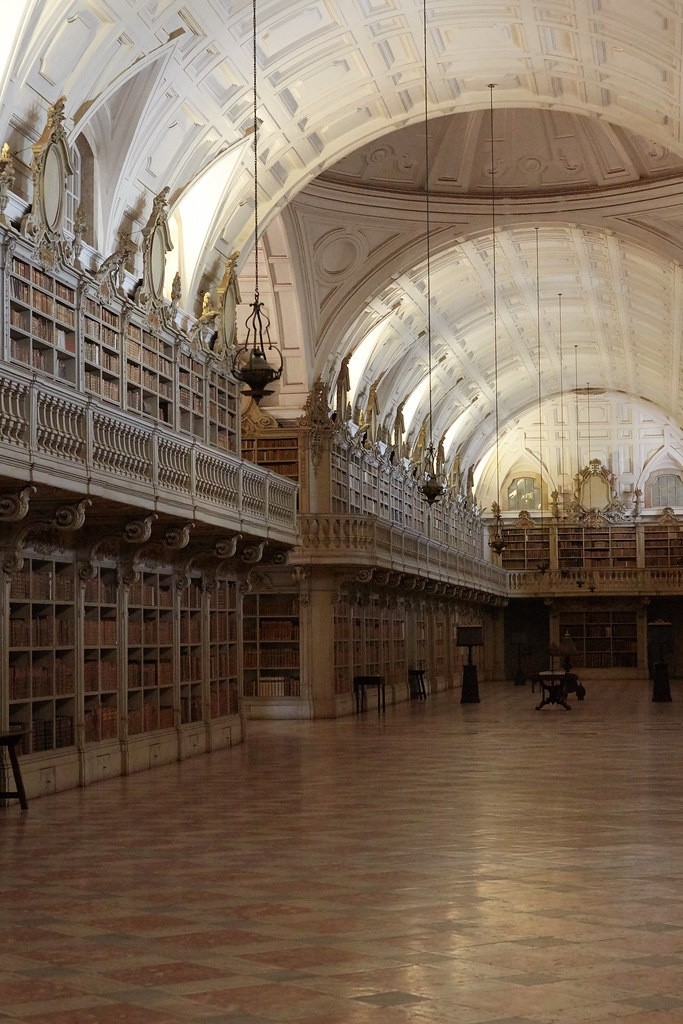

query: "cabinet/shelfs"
xmin=0 ymin=95 xmax=302 ymax=809
xmin=239 ymin=353 xmax=683 ymax=719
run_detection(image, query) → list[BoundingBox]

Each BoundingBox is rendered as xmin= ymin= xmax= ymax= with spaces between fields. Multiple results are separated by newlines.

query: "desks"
xmin=408 ymin=670 xmax=427 ymax=700
xmin=0 ymin=731 xmax=28 ymax=810
xmin=353 ymin=677 xmax=386 ymax=714
